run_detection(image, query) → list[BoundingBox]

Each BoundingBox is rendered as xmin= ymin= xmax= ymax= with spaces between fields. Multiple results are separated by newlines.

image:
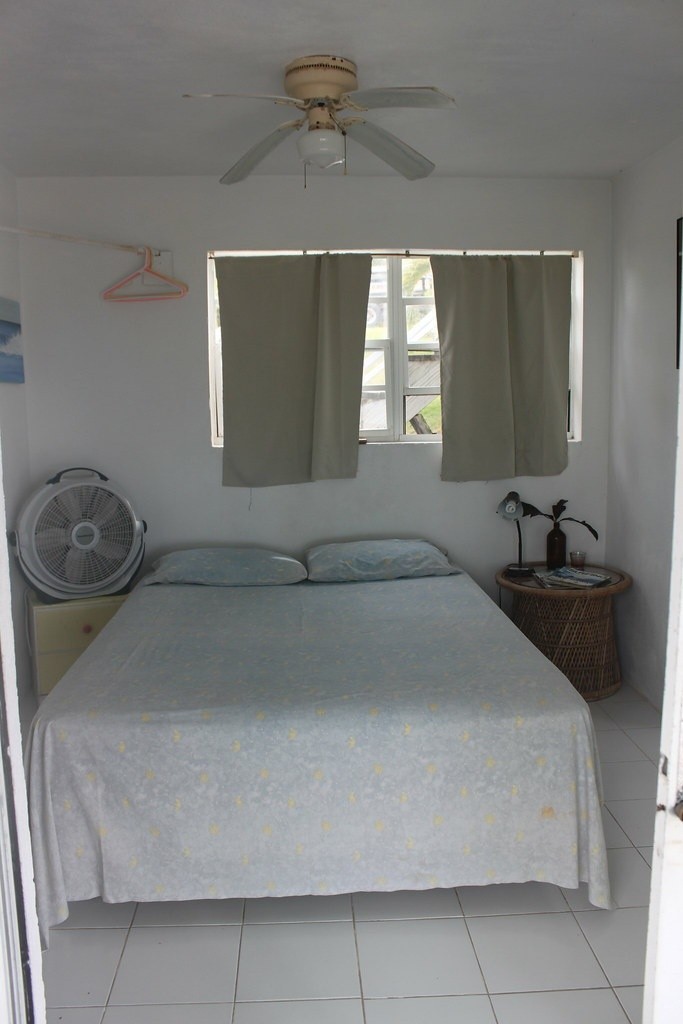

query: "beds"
xmin=22 ymin=565 xmax=613 ymax=928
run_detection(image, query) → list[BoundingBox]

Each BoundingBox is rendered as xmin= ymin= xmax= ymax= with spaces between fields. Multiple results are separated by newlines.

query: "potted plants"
xmin=517 ymin=498 xmax=599 ymax=572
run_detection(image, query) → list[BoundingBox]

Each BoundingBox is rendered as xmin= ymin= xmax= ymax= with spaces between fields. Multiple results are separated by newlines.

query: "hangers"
xmin=101 ymin=246 xmax=189 ymax=302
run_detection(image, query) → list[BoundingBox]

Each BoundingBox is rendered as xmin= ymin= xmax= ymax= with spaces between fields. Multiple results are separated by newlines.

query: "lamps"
xmin=496 ymin=491 xmax=534 ymax=577
xmin=294 ymin=126 xmax=346 ymax=172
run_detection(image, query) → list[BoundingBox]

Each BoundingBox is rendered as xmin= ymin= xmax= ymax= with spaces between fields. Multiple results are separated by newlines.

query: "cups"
xmin=570 ymin=551 xmax=586 ymax=571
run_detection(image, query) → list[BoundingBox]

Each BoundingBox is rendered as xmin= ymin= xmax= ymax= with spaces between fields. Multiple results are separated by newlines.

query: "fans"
xmin=9 ymin=466 xmax=149 ymax=604
xmin=180 ymin=53 xmax=457 ymax=186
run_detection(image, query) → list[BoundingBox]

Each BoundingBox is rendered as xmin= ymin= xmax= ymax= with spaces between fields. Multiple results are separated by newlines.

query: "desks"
xmin=497 ymin=562 xmax=633 ymax=703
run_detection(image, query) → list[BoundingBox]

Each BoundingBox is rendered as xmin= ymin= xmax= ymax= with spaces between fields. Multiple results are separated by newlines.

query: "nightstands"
xmin=22 ymin=586 xmax=130 ymax=707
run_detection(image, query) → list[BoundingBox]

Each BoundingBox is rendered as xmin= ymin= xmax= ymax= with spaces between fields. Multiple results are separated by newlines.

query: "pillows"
xmin=145 ymin=547 xmax=309 ymax=586
xmin=305 ymin=538 xmax=464 ymax=583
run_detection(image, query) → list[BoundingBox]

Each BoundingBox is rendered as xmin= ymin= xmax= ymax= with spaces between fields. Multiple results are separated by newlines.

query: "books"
xmin=533 ymin=564 xmax=612 ymax=591
